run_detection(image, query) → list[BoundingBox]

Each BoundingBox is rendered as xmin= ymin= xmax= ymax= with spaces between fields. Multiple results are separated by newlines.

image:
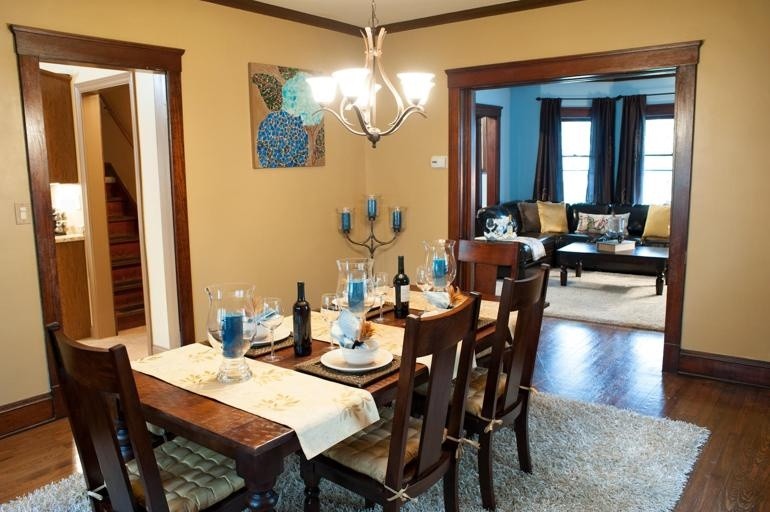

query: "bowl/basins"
xmin=339 ymin=339 xmax=383 ymax=364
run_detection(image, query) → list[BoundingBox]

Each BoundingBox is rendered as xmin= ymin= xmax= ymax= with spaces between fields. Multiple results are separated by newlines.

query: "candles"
xmin=433 ymin=258 xmax=446 ymax=284
xmin=393 ymin=210 xmax=401 ymax=231
xmin=342 ymin=212 xmax=351 ymax=233
xmin=221 ymin=310 xmax=245 ymax=357
xmin=348 ymin=280 xmax=365 ymax=312
xmin=367 ymin=196 xmax=376 ymax=217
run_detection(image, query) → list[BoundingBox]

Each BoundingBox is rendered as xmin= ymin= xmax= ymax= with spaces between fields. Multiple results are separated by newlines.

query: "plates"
xmin=215 ymin=317 xmax=292 ymax=345
xmin=321 ymin=345 xmax=394 ymax=373
xmin=421 ymin=310 xmax=449 ymax=317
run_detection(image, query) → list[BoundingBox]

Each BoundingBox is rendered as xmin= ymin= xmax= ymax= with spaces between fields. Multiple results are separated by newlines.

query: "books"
xmin=597 ymin=240 xmax=635 ymax=254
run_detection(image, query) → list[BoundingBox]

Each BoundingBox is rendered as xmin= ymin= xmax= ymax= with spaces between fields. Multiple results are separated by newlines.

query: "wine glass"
xmin=205 ymin=284 xmax=255 ymax=383
xmin=255 ymin=296 xmax=286 ymax=364
xmin=486 ymin=218 xmax=506 ymax=235
xmin=320 ymin=258 xmax=388 ymax=347
xmin=416 ymin=240 xmax=457 ymax=308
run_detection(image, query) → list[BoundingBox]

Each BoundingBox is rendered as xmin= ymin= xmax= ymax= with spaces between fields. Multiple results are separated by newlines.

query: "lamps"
xmin=305 ymin=0 xmax=437 ymax=148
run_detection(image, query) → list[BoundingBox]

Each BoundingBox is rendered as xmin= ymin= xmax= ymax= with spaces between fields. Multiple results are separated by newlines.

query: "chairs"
xmin=44 ymin=321 xmax=250 ymax=510
xmin=447 ymin=239 xmax=523 ymax=358
xmin=293 ymin=289 xmax=483 ymax=511
xmin=407 ymin=262 xmax=552 ymax=512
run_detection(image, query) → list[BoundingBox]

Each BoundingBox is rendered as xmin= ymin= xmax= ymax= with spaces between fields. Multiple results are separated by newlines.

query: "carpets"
xmin=495 ymin=267 xmax=668 ymax=333
xmin=0 ymin=390 xmax=708 ymax=512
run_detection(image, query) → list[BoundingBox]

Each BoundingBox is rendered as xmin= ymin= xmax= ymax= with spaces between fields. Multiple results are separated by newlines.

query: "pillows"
xmin=517 ymin=200 xmax=670 ymax=241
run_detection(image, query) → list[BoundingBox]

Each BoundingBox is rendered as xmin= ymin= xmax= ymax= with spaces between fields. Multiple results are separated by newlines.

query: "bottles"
xmin=292 ymin=282 xmax=313 ymax=358
xmin=393 ymin=255 xmax=411 ymax=320
xmin=506 ymin=214 xmax=516 ymax=237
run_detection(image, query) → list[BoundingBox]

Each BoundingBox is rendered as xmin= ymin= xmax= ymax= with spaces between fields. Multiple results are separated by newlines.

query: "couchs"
xmin=477 ymin=198 xmax=670 ymax=286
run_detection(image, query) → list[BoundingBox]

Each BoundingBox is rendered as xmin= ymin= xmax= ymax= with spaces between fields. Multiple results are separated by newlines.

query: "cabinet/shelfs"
xmin=55 ymin=234 xmax=92 ymax=340
xmin=40 ymin=66 xmax=78 ymax=185
